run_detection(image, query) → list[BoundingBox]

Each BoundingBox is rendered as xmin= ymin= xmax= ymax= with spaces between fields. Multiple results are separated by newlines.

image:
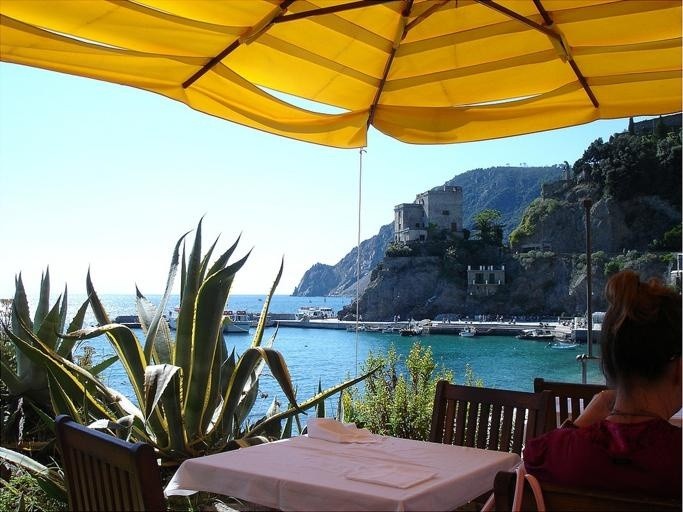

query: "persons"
xmin=522 ymin=269 xmax=680 ymax=511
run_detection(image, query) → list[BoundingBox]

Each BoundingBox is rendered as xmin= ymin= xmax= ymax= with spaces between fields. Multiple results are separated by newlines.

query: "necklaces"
xmin=605 ymin=410 xmax=666 ymax=421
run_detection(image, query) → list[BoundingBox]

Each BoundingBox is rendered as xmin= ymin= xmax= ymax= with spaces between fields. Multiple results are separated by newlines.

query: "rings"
xmin=601 ymin=390 xmax=604 ymax=392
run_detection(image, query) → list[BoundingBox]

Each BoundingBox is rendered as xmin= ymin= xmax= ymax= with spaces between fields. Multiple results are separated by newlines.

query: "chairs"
xmin=493 ymin=470 xmax=682 ymax=511
xmin=533 ymin=377 xmax=608 ymax=432
xmin=53 ymin=414 xmax=165 ymax=512
xmin=429 ymin=379 xmax=553 ymax=512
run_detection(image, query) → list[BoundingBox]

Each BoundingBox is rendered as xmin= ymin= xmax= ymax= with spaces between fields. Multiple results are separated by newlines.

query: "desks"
xmin=185 ymin=433 xmax=521 ymax=512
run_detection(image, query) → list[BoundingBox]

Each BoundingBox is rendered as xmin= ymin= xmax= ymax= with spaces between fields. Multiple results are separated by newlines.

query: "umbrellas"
xmin=0 ymin=0 xmax=682 ymax=150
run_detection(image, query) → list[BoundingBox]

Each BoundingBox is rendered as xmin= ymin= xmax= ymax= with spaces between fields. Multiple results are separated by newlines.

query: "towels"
xmin=307 ymin=418 xmax=387 ymax=443
xmin=345 ymin=465 xmax=438 ymax=489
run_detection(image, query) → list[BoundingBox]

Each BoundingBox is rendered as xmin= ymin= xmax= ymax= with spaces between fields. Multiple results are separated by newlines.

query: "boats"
xmin=111 ymin=308 xmax=274 ymax=333
xmin=458 ymin=325 xmax=477 ymax=337
xmin=346 ymin=319 xmax=423 ymax=337
xmin=515 ymin=329 xmax=556 ymax=339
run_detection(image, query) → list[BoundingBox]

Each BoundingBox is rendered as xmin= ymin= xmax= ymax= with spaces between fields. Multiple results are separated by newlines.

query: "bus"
xmin=298 ymin=307 xmax=334 ymax=319
